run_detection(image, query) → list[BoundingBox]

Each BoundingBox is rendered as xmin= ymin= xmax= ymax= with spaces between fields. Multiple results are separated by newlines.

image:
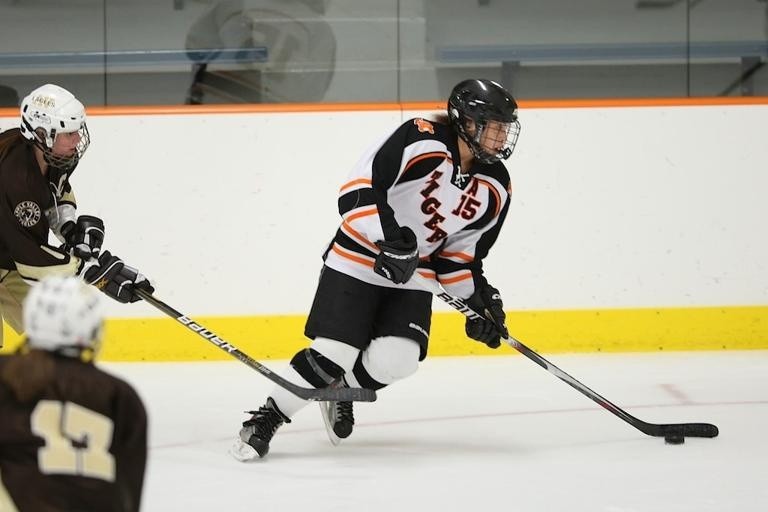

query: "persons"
xmin=184 ymin=0 xmax=337 ymax=106
xmin=237 ymin=79 xmax=521 ymax=456
xmin=0 ymin=83 xmax=154 ymax=351
xmin=0 ymin=273 xmax=146 ymax=512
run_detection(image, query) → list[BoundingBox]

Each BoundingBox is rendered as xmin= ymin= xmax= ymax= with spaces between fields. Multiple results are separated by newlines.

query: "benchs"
xmin=433 ymin=36 xmax=767 ymax=101
xmin=1 ymin=44 xmax=270 ymax=106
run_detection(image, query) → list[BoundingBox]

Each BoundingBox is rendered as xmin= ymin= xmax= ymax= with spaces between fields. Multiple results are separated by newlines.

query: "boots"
xmin=238 ymin=396 xmax=293 ymax=459
xmin=325 ymin=373 xmax=355 ymax=439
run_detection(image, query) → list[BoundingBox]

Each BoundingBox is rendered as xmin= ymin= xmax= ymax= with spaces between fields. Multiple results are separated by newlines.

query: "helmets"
xmin=446 ymin=77 xmax=522 ymax=167
xmin=22 ymin=272 xmax=104 ymax=364
xmin=19 ymin=82 xmax=91 ymax=171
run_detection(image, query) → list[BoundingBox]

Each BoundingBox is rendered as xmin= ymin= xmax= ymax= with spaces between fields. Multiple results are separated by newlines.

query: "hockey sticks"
xmin=64 ymin=246 xmax=377 ymax=401
xmin=410 ymin=272 xmax=719 ymax=439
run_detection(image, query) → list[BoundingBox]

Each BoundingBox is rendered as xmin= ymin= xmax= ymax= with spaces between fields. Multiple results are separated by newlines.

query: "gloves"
xmin=75 ymin=249 xmax=154 ymax=305
xmin=465 ymin=284 xmax=510 ymax=349
xmin=373 ymin=225 xmax=420 ymax=284
xmin=58 ymin=214 xmax=106 ymax=261
